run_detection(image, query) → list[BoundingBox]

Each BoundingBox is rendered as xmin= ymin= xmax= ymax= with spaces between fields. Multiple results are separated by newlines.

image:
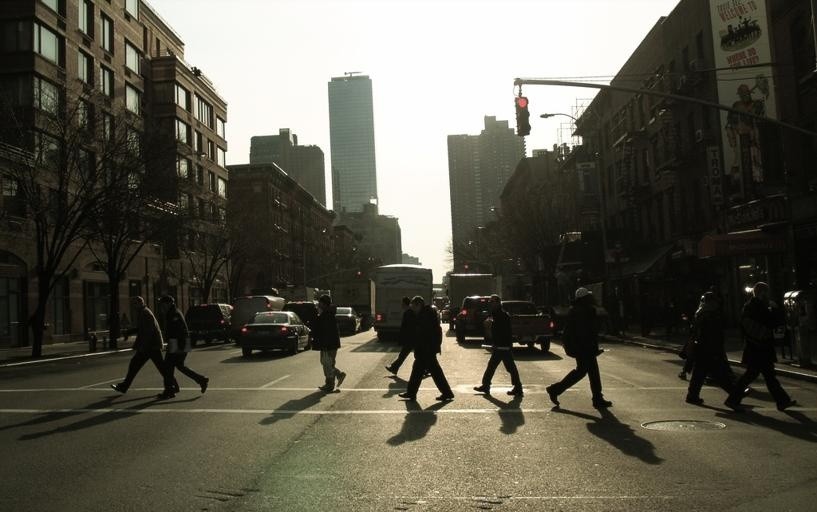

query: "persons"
xmin=473 ymin=294 xmax=524 ymax=396
xmin=545 ymin=287 xmax=612 ymax=406
xmin=685 ymin=290 xmax=750 ymax=405
xmin=109 ymin=295 xmax=180 ymax=395
xmin=424 ymin=304 xmax=442 ymax=376
xmin=398 ymin=295 xmax=455 ymax=401
xmin=678 ymin=336 xmax=699 ymax=380
xmin=311 ymin=295 xmax=346 ymax=390
xmin=726 ymin=83 xmax=770 ymax=168
xmin=723 ymin=281 xmax=797 ymax=411
xmin=156 ymin=294 xmax=208 ymax=399
xmin=121 ymin=312 xmax=130 ymax=341
xmin=385 ymin=295 xmax=431 ymax=378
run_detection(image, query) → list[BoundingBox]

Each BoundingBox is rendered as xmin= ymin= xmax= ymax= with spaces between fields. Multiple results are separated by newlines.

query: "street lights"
xmin=540 ymin=113 xmax=577 ymax=122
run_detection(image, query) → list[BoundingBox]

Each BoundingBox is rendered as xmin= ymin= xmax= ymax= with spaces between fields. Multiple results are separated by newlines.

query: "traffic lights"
xmin=516 ymin=97 xmax=530 ymax=135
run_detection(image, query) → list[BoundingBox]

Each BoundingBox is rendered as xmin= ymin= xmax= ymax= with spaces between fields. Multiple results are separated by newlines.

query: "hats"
xmin=410 ymin=296 xmax=424 ymax=303
xmin=320 ymin=294 xmax=330 ymax=305
xmin=129 ymin=296 xmax=144 ymax=305
xmin=574 ymin=287 xmax=592 ymax=298
xmin=156 ymin=296 xmax=175 ymax=303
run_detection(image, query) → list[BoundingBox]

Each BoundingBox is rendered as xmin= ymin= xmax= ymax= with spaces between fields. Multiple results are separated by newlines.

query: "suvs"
xmin=186 ymin=304 xmax=233 ymax=344
xmin=280 ymin=301 xmax=319 ymax=335
xmin=454 ymin=296 xmax=497 ymax=342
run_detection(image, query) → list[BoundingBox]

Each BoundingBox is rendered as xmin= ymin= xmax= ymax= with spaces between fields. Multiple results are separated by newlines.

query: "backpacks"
xmin=562 ymin=310 xmax=578 ymax=357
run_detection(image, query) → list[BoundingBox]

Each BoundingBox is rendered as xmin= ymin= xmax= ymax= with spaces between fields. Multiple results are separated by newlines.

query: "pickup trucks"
xmin=500 ymin=300 xmax=558 ymax=351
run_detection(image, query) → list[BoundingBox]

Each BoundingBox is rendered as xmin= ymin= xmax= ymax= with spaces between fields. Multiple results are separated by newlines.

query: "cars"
xmin=335 ymin=307 xmax=361 ymax=335
xmin=433 ymin=296 xmax=450 ymax=323
xmin=239 ymin=311 xmax=312 ymax=357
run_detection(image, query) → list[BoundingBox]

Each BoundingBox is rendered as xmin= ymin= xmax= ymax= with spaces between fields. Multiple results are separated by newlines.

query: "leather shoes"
xmin=743 ymin=388 xmax=751 ymax=396
xmin=685 ymin=399 xmax=703 ymax=405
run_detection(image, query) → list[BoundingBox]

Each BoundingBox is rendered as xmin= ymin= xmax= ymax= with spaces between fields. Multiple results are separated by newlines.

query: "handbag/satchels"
xmin=432 ymin=327 xmax=441 ymax=352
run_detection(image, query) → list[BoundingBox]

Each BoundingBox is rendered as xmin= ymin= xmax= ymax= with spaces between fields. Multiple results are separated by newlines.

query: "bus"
xmin=374 ymin=264 xmax=432 ymax=339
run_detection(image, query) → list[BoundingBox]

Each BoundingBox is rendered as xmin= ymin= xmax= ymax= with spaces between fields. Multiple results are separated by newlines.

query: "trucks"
xmin=449 ymin=274 xmax=497 ymax=331
xmin=230 ymin=297 xmax=285 ymax=345
xmin=332 ymin=276 xmax=375 ymax=331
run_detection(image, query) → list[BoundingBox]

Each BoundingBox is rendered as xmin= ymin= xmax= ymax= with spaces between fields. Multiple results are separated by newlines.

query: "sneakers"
xmin=385 ymin=366 xmax=396 ymax=374
xmin=473 ymin=386 xmax=489 ymax=392
xmin=398 ymin=392 xmax=415 ymax=398
xmin=319 ymin=385 xmax=334 ymax=391
xmin=436 ymin=392 xmax=453 ymax=400
xmin=724 ymin=400 xmax=743 ymax=410
xmin=546 ymin=386 xmax=559 ymax=405
xmin=199 ymin=377 xmax=208 ymax=393
xmin=111 ymin=384 xmax=120 ymax=391
xmin=161 ymin=393 xmax=175 ymax=399
xmin=507 ymin=390 xmax=522 ymax=395
xmin=678 ymin=372 xmax=686 ymax=380
xmin=337 ymin=373 xmax=345 ymax=387
xmin=593 ymin=398 xmax=611 ymax=407
xmin=777 ymin=400 xmax=796 ymax=410
